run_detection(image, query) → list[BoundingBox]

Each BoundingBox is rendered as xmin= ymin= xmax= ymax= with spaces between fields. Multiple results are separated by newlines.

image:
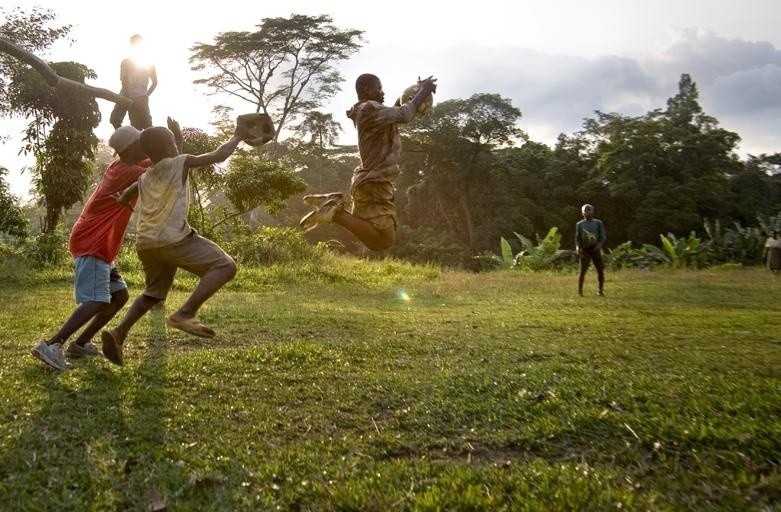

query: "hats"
xmin=109 ymin=126 xmax=144 ymax=157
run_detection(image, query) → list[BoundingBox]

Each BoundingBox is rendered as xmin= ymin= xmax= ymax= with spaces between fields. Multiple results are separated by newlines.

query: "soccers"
xmin=236 ymin=114 xmax=275 ymax=146
xmin=401 ymin=85 xmax=433 ymax=117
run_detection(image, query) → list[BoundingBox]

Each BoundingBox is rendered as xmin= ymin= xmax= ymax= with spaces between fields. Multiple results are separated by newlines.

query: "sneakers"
xmin=65 ymin=341 xmax=103 ymax=356
xmin=299 ymin=198 xmax=340 ymax=233
xmin=30 ymin=339 xmax=74 ymax=370
xmin=100 ymin=329 xmax=124 ymax=366
xmin=166 ymin=312 xmax=216 ymax=339
xmin=303 ymin=192 xmax=345 ymax=211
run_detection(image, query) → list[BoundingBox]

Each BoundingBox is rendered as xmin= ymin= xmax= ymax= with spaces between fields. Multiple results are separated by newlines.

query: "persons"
xmin=300 ymin=74 xmax=437 ymax=252
xmin=101 ymin=128 xmax=243 ymax=366
xmin=762 ymin=230 xmax=781 ymax=272
xmin=31 ymin=117 xmax=184 ymax=371
xmin=575 ymin=204 xmax=606 ymax=296
xmin=109 ymin=35 xmax=157 ymax=131
